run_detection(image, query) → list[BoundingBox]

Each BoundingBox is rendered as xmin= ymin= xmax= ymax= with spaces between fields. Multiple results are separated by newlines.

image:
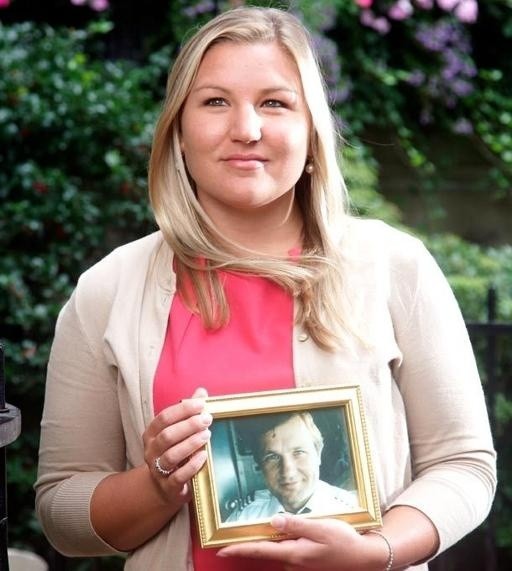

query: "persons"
xmin=222 ymin=412 xmax=360 ymax=525
xmin=30 ymin=7 xmax=499 ymax=571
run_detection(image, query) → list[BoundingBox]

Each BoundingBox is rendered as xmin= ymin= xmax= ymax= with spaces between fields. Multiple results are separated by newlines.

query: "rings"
xmin=155 ymin=457 xmax=174 ymax=476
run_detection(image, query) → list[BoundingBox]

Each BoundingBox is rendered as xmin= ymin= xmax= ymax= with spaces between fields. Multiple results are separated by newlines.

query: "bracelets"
xmin=368 ymin=529 xmax=393 ymax=571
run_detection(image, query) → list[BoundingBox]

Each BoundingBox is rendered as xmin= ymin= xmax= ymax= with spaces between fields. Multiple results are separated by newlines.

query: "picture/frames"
xmin=177 ymin=385 xmax=383 ymax=548
